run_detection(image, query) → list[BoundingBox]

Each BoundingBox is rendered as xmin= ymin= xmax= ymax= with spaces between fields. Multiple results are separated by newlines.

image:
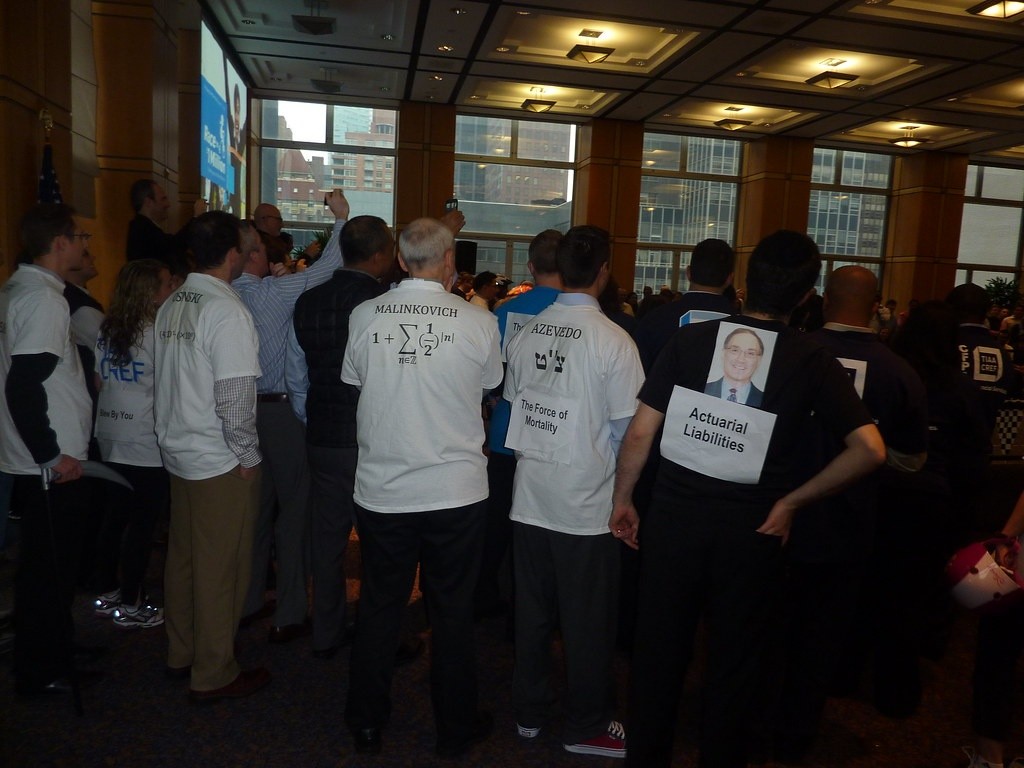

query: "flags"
xmin=38 ymin=142 xmax=64 ymax=202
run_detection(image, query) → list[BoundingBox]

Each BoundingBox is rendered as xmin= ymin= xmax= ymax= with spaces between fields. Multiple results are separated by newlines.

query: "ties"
xmin=727 ymin=389 xmax=737 ymax=403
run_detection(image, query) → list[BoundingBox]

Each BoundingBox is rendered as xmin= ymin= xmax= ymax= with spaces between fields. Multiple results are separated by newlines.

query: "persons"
xmin=89 ymin=258 xmax=177 ymax=628
xmin=126 ymin=179 xmax=207 ymax=263
xmin=798 ymin=265 xmax=931 ymax=695
xmin=293 ymin=215 xmax=426 ymax=665
xmin=876 ymin=299 xmax=993 ymax=715
xmin=232 ymin=189 xmax=349 ymax=642
xmin=1 ymin=203 xmax=107 ymax=703
xmin=704 ymin=328 xmax=764 ymax=409
xmin=472 ymin=228 xmax=563 ymax=642
xmin=634 ymin=239 xmax=735 ymax=367
xmin=502 ymin=223 xmax=647 ymax=757
xmin=223 ymin=51 xmax=247 ymax=218
xmin=280 ymin=232 xmax=1024 ymax=409
xmin=340 ymin=217 xmax=504 ymax=760
xmin=611 ymin=230 xmax=886 ymax=768
xmin=154 ymin=210 xmax=273 ymax=701
xmin=63 ymin=249 xmax=129 ymax=596
xmin=968 ymin=489 xmax=1024 ymax=768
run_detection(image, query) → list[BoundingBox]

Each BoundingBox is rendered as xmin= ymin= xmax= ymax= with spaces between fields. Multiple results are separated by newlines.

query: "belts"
xmin=256 ymin=394 xmax=289 ymax=403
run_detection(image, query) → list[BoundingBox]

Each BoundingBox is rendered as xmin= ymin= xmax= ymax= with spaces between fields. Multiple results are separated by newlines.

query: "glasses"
xmin=724 ymin=346 xmax=760 ymax=358
xmin=65 ymin=232 xmax=91 ymax=242
xmin=262 ymin=215 xmax=283 ymax=222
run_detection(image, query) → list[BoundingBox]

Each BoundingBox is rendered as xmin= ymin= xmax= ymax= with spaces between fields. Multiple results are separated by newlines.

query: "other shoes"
xmin=69 ymin=643 xmax=105 ymax=665
xmin=41 ymin=668 xmax=103 ymax=695
xmin=312 ymin=621 xmax=357 ymax=660
xmin=393 ymin=638 xmax=424 ymax=666
xmin=504 ymin=624 xmax=518 ymax=646
xmin=470 ymin=598 xmax=508 ymax=624
xmin=916 ymin=629 xmax=946 ymax=656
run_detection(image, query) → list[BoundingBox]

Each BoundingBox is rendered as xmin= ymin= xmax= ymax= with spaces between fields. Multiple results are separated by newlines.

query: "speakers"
xmin=455 ymin=241 xmax=477 ymax=276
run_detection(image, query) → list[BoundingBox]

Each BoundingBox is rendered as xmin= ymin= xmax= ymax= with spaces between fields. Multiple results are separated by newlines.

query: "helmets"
xmin=944 ymin=533 xmax=1024 ymax=616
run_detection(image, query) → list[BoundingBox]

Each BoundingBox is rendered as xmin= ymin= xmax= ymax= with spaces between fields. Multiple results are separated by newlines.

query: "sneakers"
xmin=561 ymin=720 xmax=629 ymax=760
xmin=95 ymin=585 xmax=137 ymax=619
xmin=515 ymin=720 xmax=547 ymax=738
xmin=113 ymin=601 xmax=165 ymax=629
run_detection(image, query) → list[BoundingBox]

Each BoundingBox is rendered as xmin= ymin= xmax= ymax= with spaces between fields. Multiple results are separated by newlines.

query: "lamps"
xmin=804 ymin=59 xmax=858 ymax=89
xmin=312 ymin=66 xmax=342 ymax=92
xmin=521 ymin=86 xmax=556 ymax=112
xmin=566 ymin=29 xmax=616 ymax=64
xmin=891 ymin=126 xmax=929 ymax=148
xmin=715 ymin=106 xmax=752 ymax=132
xmin=965 ymin=0 xmax=1024 ymax=18
xmin=289 ymin=0 xmax=336 ymax=37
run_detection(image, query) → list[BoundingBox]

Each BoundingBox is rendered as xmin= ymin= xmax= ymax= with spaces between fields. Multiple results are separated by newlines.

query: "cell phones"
xmin=324 ymin=193 xmax=333 ymax=205
xmin=446 ymin=199 xmax=458 ymax=212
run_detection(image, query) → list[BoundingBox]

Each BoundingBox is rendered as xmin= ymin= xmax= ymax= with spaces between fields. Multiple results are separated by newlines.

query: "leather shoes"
xmin=436 ymin=708 xmax=493 ymax=760
xmin=166 ymin=664 xmax=191 ymax=680
xmin=191 ymin=668 xmax=272 ymax=704
xmin=237 ymin=600 xmax=275 ymax=631
xmin=352 ymin=726 xmax=381 ymax=756
xmin=269 ymin=620 xmax=312 ymax=643
